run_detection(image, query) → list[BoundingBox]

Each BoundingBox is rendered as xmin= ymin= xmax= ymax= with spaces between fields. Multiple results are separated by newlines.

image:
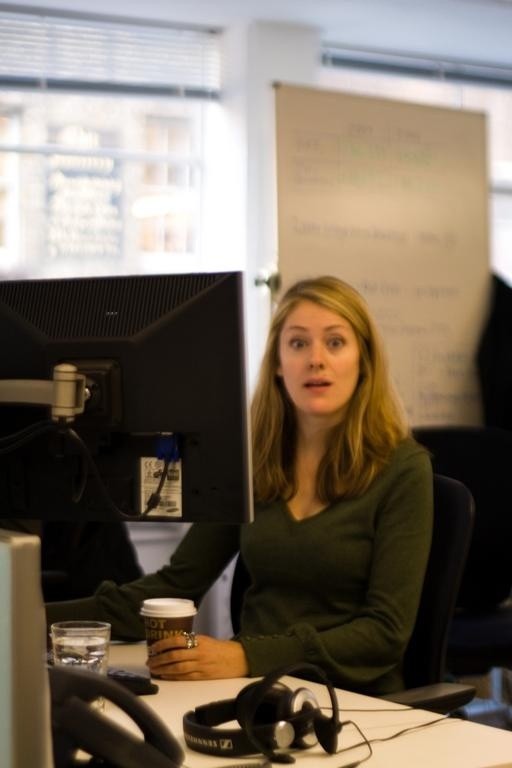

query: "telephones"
xmin=47 ymin=664 xmax=184 ymax=768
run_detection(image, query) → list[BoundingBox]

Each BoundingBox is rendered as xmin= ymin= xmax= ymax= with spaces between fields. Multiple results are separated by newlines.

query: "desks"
xmin=71 ymin=635 xmax=512 ymax=768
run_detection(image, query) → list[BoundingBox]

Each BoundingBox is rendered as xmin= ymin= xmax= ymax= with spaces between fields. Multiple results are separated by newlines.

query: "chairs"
xmin=380 ymin=469 xmax=475 ymax=718
xmin=412 ymin=426 xmax=511 ymax=677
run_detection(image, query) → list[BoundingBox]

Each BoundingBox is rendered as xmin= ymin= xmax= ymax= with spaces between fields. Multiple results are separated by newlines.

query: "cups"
xmin=52 ymin=619 xmax=110 ymax=718
xmin=141 ymin=597 xmax=196 ymax=680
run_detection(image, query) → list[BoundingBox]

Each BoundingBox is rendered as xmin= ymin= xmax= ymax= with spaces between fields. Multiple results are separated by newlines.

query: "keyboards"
xmin=45 ymin=643 xmax=158 ymax=696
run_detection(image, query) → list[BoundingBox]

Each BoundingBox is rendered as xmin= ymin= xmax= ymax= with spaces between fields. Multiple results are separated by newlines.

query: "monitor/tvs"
xmin=1 ymin=270 xmax=254 ymax=523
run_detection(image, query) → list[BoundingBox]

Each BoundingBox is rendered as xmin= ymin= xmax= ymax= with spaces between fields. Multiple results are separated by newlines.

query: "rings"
xmin=181 ymin=631 xmax=198 ymax=649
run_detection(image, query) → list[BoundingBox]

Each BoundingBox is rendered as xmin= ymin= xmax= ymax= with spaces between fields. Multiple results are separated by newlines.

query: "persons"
xmin=43 ymin=274 xmax=436 ymax=697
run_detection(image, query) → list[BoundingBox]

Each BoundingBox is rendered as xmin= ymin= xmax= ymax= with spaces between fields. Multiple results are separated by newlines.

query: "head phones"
xmin=183 ymin=680 xmax=320 ymax=756
xmin=246 ymin=662 xmax=341 ymax=763
